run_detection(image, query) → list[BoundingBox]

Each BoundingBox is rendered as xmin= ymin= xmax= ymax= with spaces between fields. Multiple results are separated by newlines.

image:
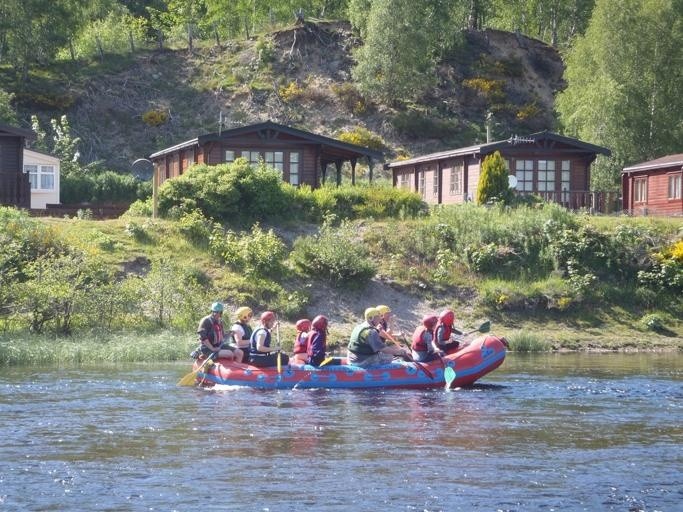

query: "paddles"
xmin=440 ymin=352 xmax=456 ymax=385
xmin=276 ymin=323 xmax=281 ymax=373
xmin=382 ymin=331 xmax=435 ymax=380
xmin=176 ymin=333 xmax=233 ymax=386
xmin=318 ymin=357 xmax=332 ymax=366
xmin=454 ymin=321 xmax=489 ymax=340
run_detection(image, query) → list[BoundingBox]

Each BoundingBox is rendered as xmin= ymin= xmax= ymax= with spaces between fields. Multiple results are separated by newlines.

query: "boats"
xmin=191 ymin=333 xmax=507 ymax=393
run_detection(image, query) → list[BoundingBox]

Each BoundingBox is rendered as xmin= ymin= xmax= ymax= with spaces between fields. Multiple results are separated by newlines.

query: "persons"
xmin=433 ymin=310 xmax=469 ymax=354
xmin=196 ymin=302 xmax=236 ymax=360
xmin=229 ymin=307 xmax=254 ymax=363
xmin=292 ymin=318 xmax=330 ymax=366
xmin=375 ymin=305 xmax=405 ymax=352
xmin=306 ymin=315 xmax=349 ymax=366
xmin=411 ymin=315 xmax=444 ymax=363
xmin=247 ymin=311 xmax=289 ymax=367
xmin=347 ymin=308 xmax=412 ymax=367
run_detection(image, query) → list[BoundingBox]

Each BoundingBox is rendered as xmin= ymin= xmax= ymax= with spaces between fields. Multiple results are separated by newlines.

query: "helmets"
xmin=365 ymin=308 xmax=383 ymax=321
xmin=296 ymin=319 xmax=311 ymax=331
xmin=261 ymin=311 xmax=275 ymax=326
xmin=423 ymin=315 xmax=438 ymax=327
xmin=313 ymin=315 xmax=328 ymax=330
xmin=439 ymin=310 xmax=453 ymax=324
xmin=376 ymin=305 xmax=391 ymax=319
xmin=211 ymin=303 xmax=224 ymax=311
xmin=236 ymin=307 xmax=252 ymax=320
